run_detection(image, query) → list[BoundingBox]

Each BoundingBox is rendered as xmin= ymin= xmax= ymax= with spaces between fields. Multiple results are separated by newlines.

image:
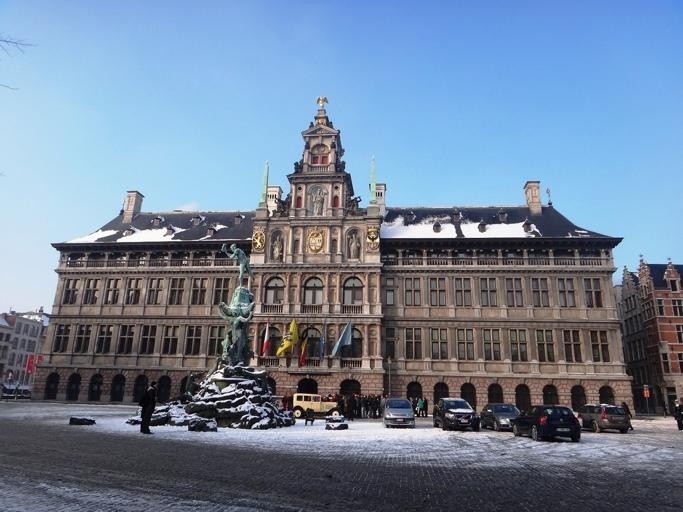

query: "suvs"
xmin=293 ymin=393 xmax=342 ymax=419
xmin=433 ymin=398 xmax=482 ymax=431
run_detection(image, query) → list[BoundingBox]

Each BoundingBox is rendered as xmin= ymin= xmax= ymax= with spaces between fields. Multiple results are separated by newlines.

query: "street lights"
xmin=388 ymin=355 xmax=393 ymax=398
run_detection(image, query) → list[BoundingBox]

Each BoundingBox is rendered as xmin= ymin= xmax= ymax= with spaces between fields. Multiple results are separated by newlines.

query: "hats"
xmin=152 ymin=380 xmax=156 ymax=385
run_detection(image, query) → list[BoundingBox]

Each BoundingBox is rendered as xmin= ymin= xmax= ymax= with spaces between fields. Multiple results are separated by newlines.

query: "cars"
xmin=578 ymin=403 xmax=630 ymax=433
xmin=480 ymin=403 xmax=521 ymax=430
xmin=384 ymin=398 xmax=415 ymax=428
xmin=512 ymin=404 xmax=581 ymax=441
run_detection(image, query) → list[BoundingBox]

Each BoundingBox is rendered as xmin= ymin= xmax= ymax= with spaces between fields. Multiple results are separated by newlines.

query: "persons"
xmin=348 ymin=234 xmax=361 ymax=258
xmin=272 ymin=235 xmax=282 ymax=260
xmin=305 ymin=408 xmax=315 ymax=425
xmin=322 ymin=391 xmax=386 ymax=421
xmin=409 ymin=396 xmax=428 ymax=417
xmin=621 ymin=402 xmax=634 ymax=430
xmin=670 ymin=396 xmax=683 ymax=430
xmin=139 ymin=381 xmax=158 ymax=434
xmin=313 ymin=188 xmax=324 ymax=216
xmin=221 ymin=243 xmax=255 ymax=286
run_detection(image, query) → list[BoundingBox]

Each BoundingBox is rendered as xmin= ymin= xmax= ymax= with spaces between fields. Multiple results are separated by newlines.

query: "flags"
xmin=276 ymin=318 xmax=299 ymax=357
xmin=331 ymin=321 xmax=352 ymax=357
xmin=262 ymin=323 xmax=270 ymax=356
xmin=318 ymin=323 xmax=326 ymax=362
xmin=298 ymin=333 xmax=309 ymax=368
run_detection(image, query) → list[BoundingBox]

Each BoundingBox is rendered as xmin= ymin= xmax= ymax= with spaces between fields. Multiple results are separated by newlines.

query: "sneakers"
xmin=140 ymin=430 xmax=152 ymax=434
